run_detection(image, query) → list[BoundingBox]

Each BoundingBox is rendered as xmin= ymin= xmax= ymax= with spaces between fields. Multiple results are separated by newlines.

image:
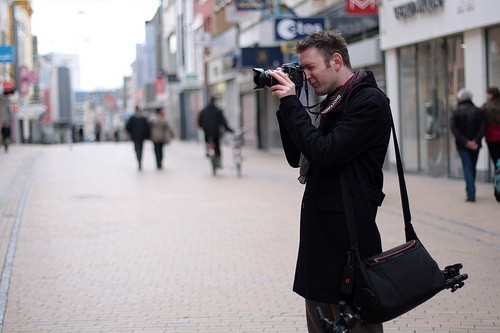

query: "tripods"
xmin=315 ymin=263 xmax=470 ymax=333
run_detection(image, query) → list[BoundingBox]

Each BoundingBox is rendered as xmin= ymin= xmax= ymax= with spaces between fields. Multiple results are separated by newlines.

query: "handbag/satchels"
xmin=353 ymin=223 xmax=447 ymax=323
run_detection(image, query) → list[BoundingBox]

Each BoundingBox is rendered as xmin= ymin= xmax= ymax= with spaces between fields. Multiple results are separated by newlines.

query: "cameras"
xmin=253 ymin=62 xmax=304 ymax=91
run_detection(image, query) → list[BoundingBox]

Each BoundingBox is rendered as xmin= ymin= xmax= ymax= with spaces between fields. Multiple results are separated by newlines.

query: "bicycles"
xmin=230 ymin=128 xmax=253 ymax=173
xmin=208 ymin=135 xmax=220 ymax=174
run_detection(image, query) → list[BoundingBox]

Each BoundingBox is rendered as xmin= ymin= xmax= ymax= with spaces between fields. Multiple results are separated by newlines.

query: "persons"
xmin=78 ymin=126 xmax=83 ymax=142
xmin=424 ymin=88 xmax=439 ymax=175
xmin=72 ymin=125 xmax=77 ymax=142
xmin=147 ymin=107 xmax=174 ymax=168
xmin=1 ymin=123 xmax=11 ymax=151
xmin=481 ymin=87 xmax=500 ymax=202
xmin=126 ymin=105 xmax=150 ymax=168
xmin=450 ymin=88 xmax=484 ymax=202
xmin=114 ymin=130 xmax=119 ymax=141
xmin=267 ymin=31 xmax=392 ymax=333
xmin=94 ymin=122 xmax=101 ymax=141
xmin=197 ymin=96 xmax=235 ymax=167
